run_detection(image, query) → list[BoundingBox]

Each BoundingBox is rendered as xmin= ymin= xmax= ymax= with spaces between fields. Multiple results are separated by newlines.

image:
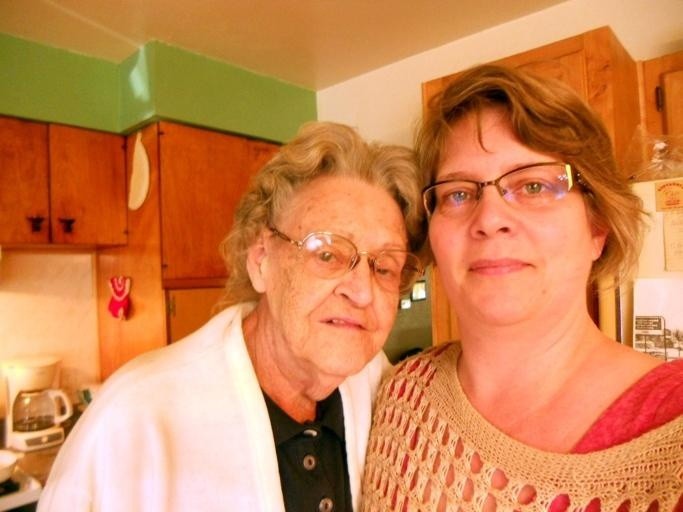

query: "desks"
xmin=1 ymin=445 xmax=63 ymax=486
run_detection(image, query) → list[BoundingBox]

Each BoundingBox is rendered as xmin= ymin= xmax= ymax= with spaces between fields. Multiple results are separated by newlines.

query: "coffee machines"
xmin=3 ymin=353 xmax=74 ymax=453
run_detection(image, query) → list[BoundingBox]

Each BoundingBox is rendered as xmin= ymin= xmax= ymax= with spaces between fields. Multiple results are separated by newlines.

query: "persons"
xmin=32 ymin=117 xmax=435 ymax=512
xmin=353 ymin=62 xmax=682 ymax=512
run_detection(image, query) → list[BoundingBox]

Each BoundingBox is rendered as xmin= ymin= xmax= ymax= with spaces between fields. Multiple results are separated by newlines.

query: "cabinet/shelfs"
xmin=0 ymin=115 xmax=126 ymax=255
xmin=95 ymin=121 xmax=286 ymax=384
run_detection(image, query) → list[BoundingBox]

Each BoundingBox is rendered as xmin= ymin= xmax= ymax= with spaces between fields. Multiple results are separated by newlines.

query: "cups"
xmin=0 ymin=448 xmax=24 ymax=485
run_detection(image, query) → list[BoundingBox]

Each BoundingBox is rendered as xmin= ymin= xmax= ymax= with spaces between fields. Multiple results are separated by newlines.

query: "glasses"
xmin=266 ymin=222 xmax=426 ymax=294
xmin=423 ymin=162 xmax=587 ymax=222
